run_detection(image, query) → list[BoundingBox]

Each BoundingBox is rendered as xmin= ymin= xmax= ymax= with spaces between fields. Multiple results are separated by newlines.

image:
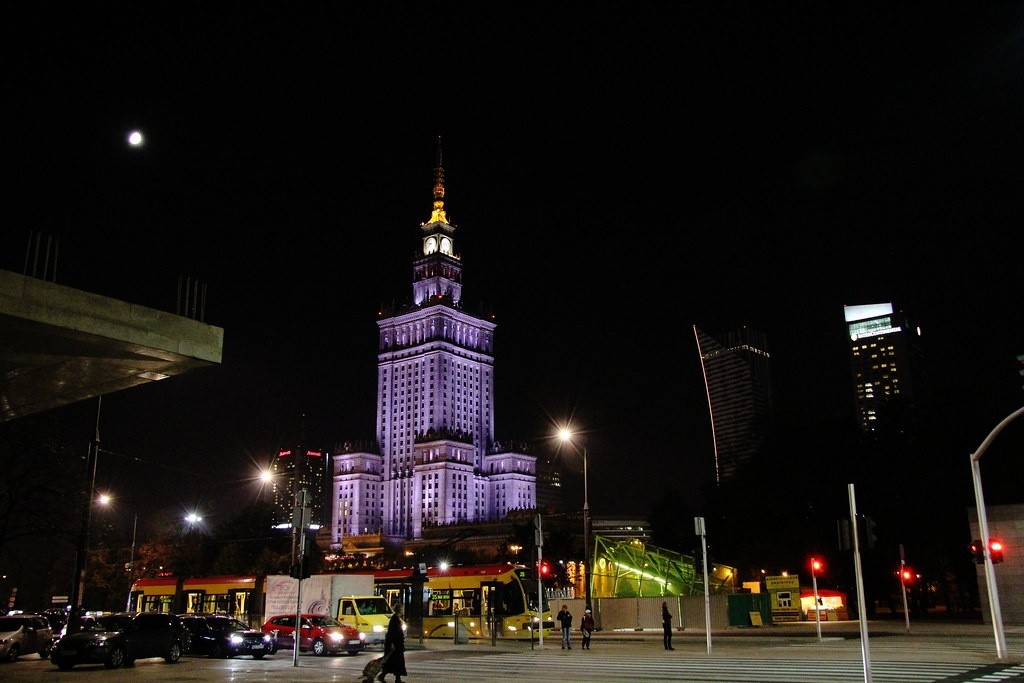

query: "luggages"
xmin=362 ymin=647 xmax=395 ymax=683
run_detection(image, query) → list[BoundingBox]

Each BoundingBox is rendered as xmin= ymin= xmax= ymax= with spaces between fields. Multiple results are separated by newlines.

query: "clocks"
xmin=425 ymin=238 xmax=437 ymax=251
xmin=441 ymin=238 xmax=450 ymax=253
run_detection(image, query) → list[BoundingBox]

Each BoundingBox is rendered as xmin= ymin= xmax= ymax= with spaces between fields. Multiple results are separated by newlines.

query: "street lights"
xmin=559 ymin=428 xmax=596 ymax=632
xmin=511 ymin=545 xmax=522 ymax=555
xmin=260 ymin=469 xmax=306 ymax=667
xmin=100 ymin=494 xmax=138 ymax=595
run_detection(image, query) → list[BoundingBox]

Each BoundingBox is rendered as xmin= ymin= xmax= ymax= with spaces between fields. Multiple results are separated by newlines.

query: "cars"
xmin=0 ymin=614 xmax=53 ymax=664
xmin=58 ymin=609 xmax=112 ymax=639
xmin=261 ymin=612 xmax=366 ymax=657
xmin=175 ymin=611 xmax=279 ymax=659
xmin=50 ymin=610 xmax=191 ymax=671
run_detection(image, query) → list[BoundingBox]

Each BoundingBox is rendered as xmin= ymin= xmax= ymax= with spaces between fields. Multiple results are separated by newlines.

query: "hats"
xmin=585 ymin=609 xmax=592 ymax=614
xmin=562 ymin=604 xmax=567 ymax=609
xmin=393 ymin=602 xmax=403 ymax=612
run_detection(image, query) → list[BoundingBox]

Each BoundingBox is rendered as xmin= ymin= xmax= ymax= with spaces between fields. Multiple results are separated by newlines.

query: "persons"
xmin=556 ymin=604 xmax=573 ymax=650
xmin=580 ymin=609 xmax=594 ymax=649
xmin=433 ymin=600 xmax=444 ymax=608
xmin=378 ymin=603 xmax=407 ymax=683
xmin=661 ymin=602 xmax=676 ymax=650
xmin=454 ymin=603 xmax=460 ymax=614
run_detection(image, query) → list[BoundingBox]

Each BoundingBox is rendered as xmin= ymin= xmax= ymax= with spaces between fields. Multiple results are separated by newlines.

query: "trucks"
xmin=264 ymin=573 xmax=407 ymax=651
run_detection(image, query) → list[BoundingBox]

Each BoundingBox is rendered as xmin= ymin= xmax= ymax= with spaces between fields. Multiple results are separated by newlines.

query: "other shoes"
xmin=665 ymin=647 xmax=675 ymax=650
xmin=568 ymin=647 xmax=572 ymax=649
xmin=586 ymin=646 xmax=589 ymax=649
xmin=583 ymin=648 xmax=585 ymax=650
xmin=562 ymin=646 xmax=565 ymax=649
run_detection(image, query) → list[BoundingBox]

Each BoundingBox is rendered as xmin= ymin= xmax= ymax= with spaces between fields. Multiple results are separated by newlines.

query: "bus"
xmin=126 ymin=564 xmax=556 ymax=640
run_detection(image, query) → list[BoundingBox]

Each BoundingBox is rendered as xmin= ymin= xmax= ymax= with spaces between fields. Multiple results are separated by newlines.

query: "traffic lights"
xmin=970 ymin=539 xmax=985 ymax=565
xmin=904 ymin=570 xmax=910 ymax=583
xmin=989 ymin=537 xmax=1004 ymax=564
xmin=542 ymin=563 xmax=548 ymax=578
xmin=814 ymin=560 xmax=820 ymax=577
